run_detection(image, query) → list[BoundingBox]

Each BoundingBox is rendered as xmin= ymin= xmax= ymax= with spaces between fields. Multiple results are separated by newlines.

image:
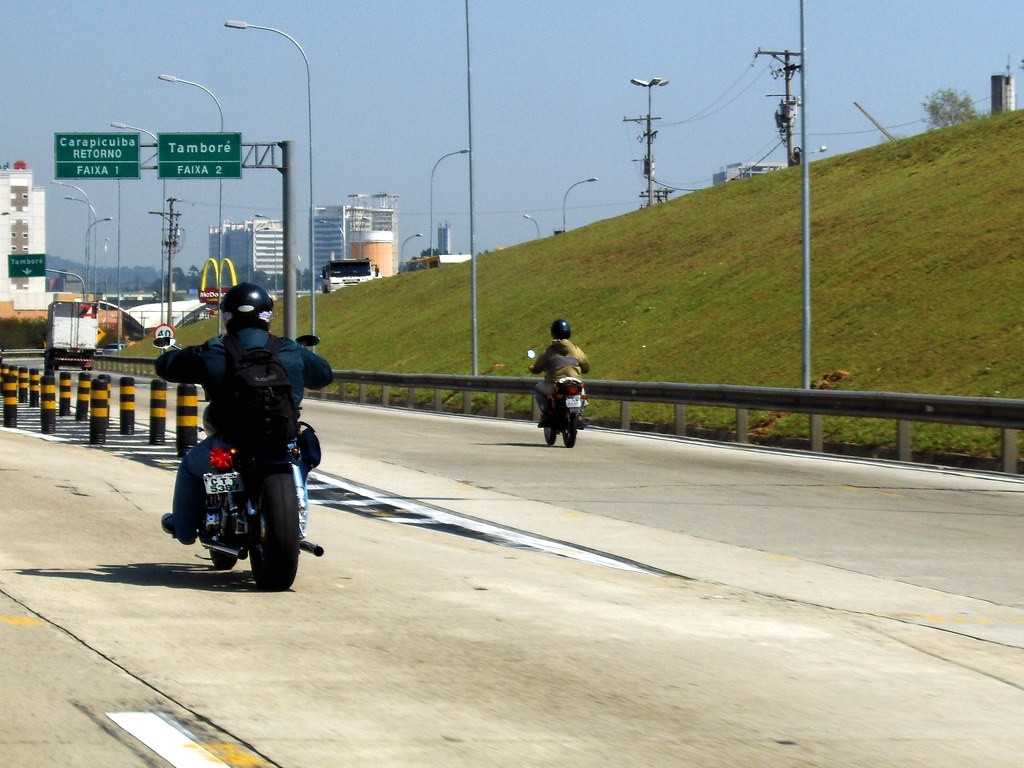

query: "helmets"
xmin=551 ymin=319 xmax=571 ymax=340
xmin=219 ymin=282 xmax=274 ymax=333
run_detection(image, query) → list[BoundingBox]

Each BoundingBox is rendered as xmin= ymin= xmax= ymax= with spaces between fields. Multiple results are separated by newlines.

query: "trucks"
xmin=321 ymin=257 xmax=374 ymax=294
xmin=42 ymin=300 xmax=97 ymax=371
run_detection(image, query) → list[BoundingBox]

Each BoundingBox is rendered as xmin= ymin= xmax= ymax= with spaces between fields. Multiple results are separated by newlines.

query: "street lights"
xmin=561 ymin=176 xmax=597 ymax=231
xmin=110 ymin=121 xmax=165 ymax=325
xmin=49 ymin=178 xmax=114 ymax=304
xmin=398 ymin=233 xmax=423 ymax=273
xmin=223 ymin=19 xmax=318 ymax=356
xmin=157 ymin=73 xmax=223 ymax=336
xmin=318 ymin=219 xmax=347 ymax=260
xmin=523 ymin=215 xmax=541 ymax=240
xmin=429 ymin=148 xmax=469 ymax=255
xmin=253 ymin=213 xmax=278 ymax=293
xmin=630 ymin=77 xmax=670 ymax=208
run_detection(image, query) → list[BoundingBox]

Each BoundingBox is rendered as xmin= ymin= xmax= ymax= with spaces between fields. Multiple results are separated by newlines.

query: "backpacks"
xmin=212 ymin=335 xmax=302 ymax=449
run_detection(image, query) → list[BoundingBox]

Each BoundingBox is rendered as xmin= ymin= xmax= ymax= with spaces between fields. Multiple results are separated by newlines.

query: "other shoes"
xmin=161 ymin=513 xmax=196 ymax=545
xmin=538 ymin=413 xmax=552 ymax=428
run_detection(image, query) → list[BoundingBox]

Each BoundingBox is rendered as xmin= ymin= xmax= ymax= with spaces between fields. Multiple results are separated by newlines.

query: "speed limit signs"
xmin=154 ymin=324 xmax=176 ymax=342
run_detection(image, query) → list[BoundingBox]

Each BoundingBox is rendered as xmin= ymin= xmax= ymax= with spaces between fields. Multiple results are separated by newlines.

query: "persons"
xmin=528 ymin=319 xmax=590 ymax=430
xmin=154 ymin=283 xmax=333 ymax=545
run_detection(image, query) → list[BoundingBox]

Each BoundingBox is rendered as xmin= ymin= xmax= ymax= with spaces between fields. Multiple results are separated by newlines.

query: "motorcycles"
xmin=527 ymin=350 xmax=590 ymax=448
xmin=151 ymin=334 xmax=324 ymax=593
